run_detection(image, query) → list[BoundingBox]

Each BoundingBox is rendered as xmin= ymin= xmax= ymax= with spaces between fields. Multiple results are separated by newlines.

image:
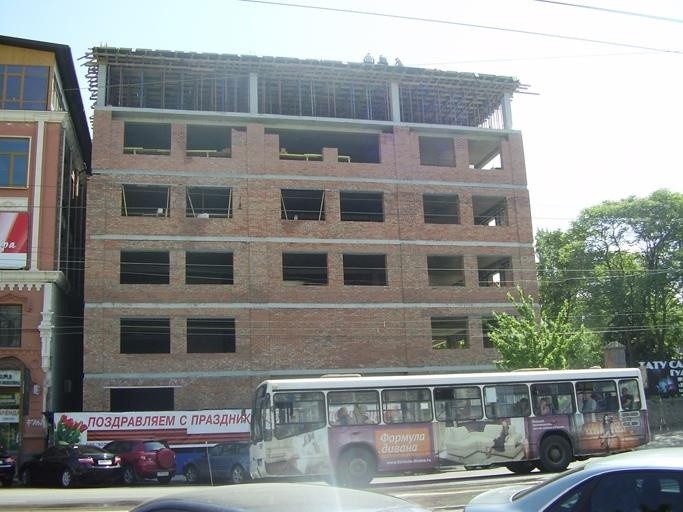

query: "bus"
xmin=248 ymin=367 xmax=651 ymax=488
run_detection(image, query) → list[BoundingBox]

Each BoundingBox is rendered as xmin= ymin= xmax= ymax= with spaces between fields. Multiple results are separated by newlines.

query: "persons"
xmin=600 ymin=415 xmax=614 ymax=454
xmin=621 ymin=386 xmax=635 ymax=411
xmin=485 ymin=418 xmax=509 ymax=458
xmin=582 ymin=389 xmax=597 ymax=413
xmin=282 ymin=432 xmax=314 ymax=472
xmin=507 ymin=398 xmax=553 ymax=416
xmin=337 ymin=407 xmax=354 ymax=425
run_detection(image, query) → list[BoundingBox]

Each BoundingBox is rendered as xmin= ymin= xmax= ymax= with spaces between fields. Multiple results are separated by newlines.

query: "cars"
xmin=461 ymin=446 xmax=683 ymax=512
xmin=17 ymin=444 xmax=124 ymax=488
xmin=127 ymin=482 xmax=434 ymax=512
xmin=180 ymin=440 xmax=251 ymax=486
xmin=0 ymin=446 xmax=17 ymax=487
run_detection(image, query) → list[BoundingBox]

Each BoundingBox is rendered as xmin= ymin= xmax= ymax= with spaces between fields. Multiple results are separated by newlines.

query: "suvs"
xmin=102 ymin=438 xmax=177 ymax=485
